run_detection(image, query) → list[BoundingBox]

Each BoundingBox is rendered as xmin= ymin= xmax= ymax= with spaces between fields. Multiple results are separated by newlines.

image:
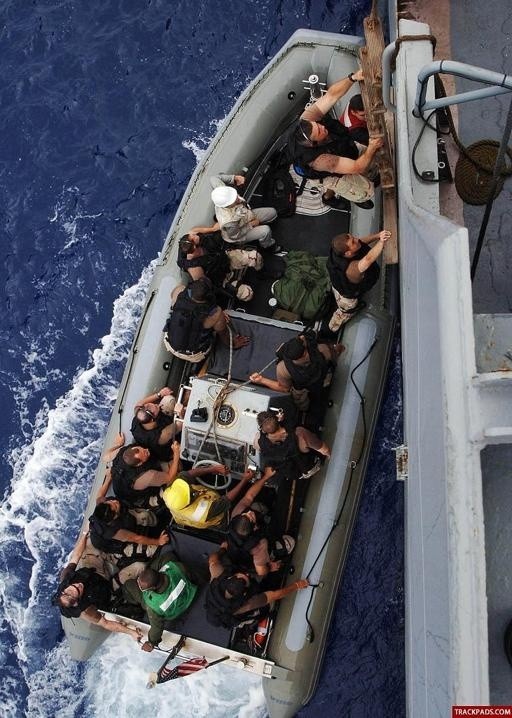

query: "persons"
xmin=288 ymin=68 xmax=383 ymax=211
xmin=208 ymin=174 xmax=282 ymax=258
xmin=52 ymin=223 xmax=391 ymax=652
xmin=322 ymin=93 xmax=369 ymax=211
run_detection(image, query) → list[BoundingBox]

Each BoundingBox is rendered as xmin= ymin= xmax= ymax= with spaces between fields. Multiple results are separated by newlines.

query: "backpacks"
xmin=262 ymin=168 xmax=296 ymax=219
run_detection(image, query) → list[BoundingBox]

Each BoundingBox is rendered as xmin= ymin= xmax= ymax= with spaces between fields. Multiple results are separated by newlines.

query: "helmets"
xmin=162 ymin=478 xmax=192 ymax=511
xmin=210 ymin=185 xmax=240 ymax=208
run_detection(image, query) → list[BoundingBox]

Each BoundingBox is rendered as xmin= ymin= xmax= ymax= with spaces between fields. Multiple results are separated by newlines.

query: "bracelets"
xmin=346 ymin=72 xmax=358 ymax=83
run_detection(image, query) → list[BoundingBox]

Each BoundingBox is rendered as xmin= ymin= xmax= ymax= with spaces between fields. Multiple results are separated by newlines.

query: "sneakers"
xmin=352 ymin=173 xmax=381 ymax=210
